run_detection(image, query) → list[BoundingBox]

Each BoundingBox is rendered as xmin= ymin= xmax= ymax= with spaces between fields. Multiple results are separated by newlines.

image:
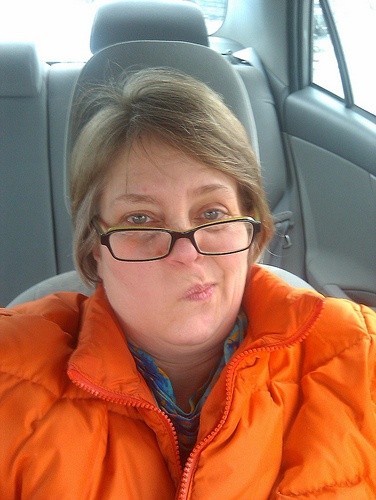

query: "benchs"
xmin=0 ymin=0 xmax=290 ymax=309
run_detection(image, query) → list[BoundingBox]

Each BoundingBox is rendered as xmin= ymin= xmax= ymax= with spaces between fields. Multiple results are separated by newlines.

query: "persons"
xmin=1 ymin=65 xmax=376 ymax=497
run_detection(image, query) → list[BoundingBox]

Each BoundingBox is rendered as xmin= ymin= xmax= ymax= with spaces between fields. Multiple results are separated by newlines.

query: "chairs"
xmin=5 ymin=40 xmax=315 ymax=309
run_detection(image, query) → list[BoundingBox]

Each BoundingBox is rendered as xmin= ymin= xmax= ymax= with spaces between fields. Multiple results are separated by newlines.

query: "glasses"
xmin=91 ymin=218 xmax=261 ymax=261
